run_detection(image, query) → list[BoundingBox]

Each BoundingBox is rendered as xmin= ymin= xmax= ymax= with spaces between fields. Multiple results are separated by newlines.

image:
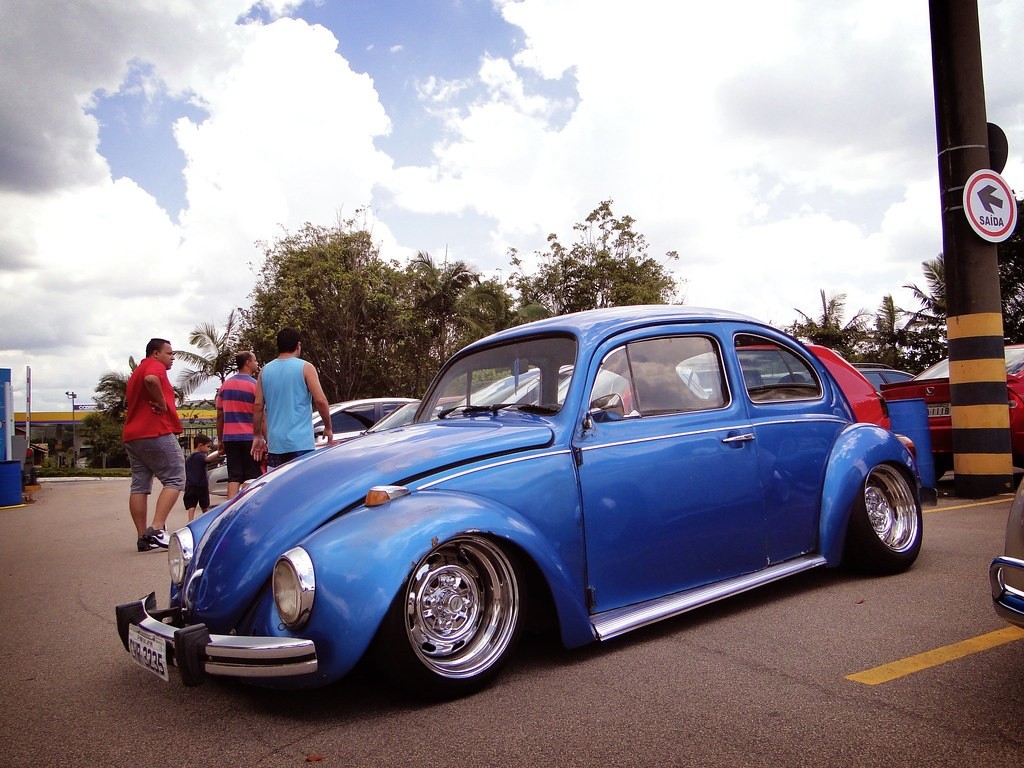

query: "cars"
xmin=204 ymin=398 xmax=422 ymax=495
xmin=908 ymin=344 xmax=1024 ymax=482
xmin=531 ymin=344 xmax=890 ymax=435
xmin=116 ymin=303 xmax=921 ymax=700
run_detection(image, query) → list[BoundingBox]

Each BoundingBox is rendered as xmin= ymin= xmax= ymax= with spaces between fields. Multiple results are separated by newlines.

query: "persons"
xmin=122 ymin=338 xmax=187 ymax=552
xmin=251 ymin=328 xmax=333 ymax=475
xmin=183 ymin=434 xmax=224 ymax=523
xmin=217 ymin=350 xmax=268 ymax=500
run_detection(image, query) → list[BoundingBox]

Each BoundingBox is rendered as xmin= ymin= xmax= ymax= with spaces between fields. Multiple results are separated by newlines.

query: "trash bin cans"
xmin=0 ymin=458 xmax=24 ymax=507
xmin=885 ymin=398 xmax=937 ymax=505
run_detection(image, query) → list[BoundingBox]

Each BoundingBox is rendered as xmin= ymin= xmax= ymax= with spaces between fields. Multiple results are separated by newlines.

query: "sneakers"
xmin=137 ymin=535 xmax=159 ymax=551
xmin=142 ymin=524 xmax=170 ymax=548
